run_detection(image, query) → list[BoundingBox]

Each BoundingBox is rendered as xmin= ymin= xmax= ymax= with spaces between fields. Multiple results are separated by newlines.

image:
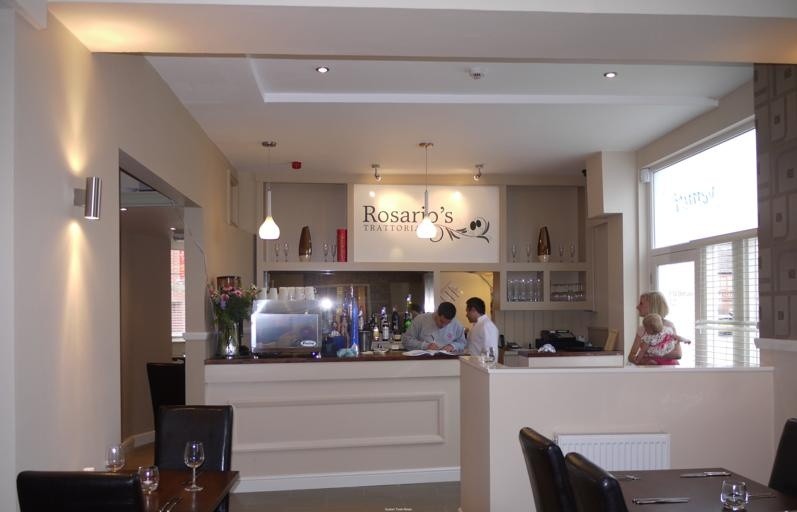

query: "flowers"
xmin=207 ymin=283 xmax=261 ymax=352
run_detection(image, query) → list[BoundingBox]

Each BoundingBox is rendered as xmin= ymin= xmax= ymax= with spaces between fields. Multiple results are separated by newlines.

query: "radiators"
xmin=553 ymin=434 xmax=671 ymax=471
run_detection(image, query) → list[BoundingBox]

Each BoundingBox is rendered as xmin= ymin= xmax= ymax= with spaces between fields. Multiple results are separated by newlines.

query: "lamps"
xmin=258 ymin=141 xmax=282 ymax=240
xmin=71 ymin=176 xmax=100 ymax=220
xmin=417 ymin=141 xmax=437 ymax=238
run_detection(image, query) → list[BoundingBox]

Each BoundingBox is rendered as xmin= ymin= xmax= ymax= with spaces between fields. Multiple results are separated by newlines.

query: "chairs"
xmin=767 ymin=418 xmax=796 ymax=498
xmin=17 ymin=363 xmax=240 ymax=511
xmin=520 ymin=425 xmax=625 ymax=509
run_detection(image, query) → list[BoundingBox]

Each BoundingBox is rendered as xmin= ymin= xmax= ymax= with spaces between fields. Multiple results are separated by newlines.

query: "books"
xmin=401 ymin=347 xmax=459 ymax=356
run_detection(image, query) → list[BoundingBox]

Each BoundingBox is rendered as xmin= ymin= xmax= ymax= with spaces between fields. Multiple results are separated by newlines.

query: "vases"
xmin=218 ymin=320 xmax=241 ymax=360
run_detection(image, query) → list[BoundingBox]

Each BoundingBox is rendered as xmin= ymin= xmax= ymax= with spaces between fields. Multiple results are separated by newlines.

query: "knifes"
xmin=633 ymin=495 xmax=691 ymax=505
xmin=676 ymin=470 xmax=733 ymax=477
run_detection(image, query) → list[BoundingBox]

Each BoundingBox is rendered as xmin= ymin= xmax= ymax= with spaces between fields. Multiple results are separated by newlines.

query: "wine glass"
xmin=508 ymin=242 xmax=578 ymax=265
xmin=549 ymin=282 xmax=585 ymax=302
xmin=103 ymin=439 xmax=205 ymax=512
xmin=273 ymin=240 xmax=337 ymax=263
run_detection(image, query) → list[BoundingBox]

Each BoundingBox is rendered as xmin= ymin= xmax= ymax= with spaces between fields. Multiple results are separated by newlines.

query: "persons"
xmin=465 ymin=297 xmax=499 ymax=364
xmin=407 ymin=303 xmax=423 ymax=319
xmin=627 ymin=290 xmax=682 ymax=366
xmin=402 ymin=301 xmax=467 ymax=353
xmin=633 ymin=313 xmax=691 ymax=365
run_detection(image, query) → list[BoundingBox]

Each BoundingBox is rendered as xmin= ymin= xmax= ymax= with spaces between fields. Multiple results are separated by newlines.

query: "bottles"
xmin=328 ymin=284 xmax=413 ymax=343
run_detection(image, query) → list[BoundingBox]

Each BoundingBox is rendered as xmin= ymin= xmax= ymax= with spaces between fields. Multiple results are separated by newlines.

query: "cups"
xmin=720 ymin=479 xmax=749 ymax=512
xmin=505 ymin=278 xmax=544 ymax=302
